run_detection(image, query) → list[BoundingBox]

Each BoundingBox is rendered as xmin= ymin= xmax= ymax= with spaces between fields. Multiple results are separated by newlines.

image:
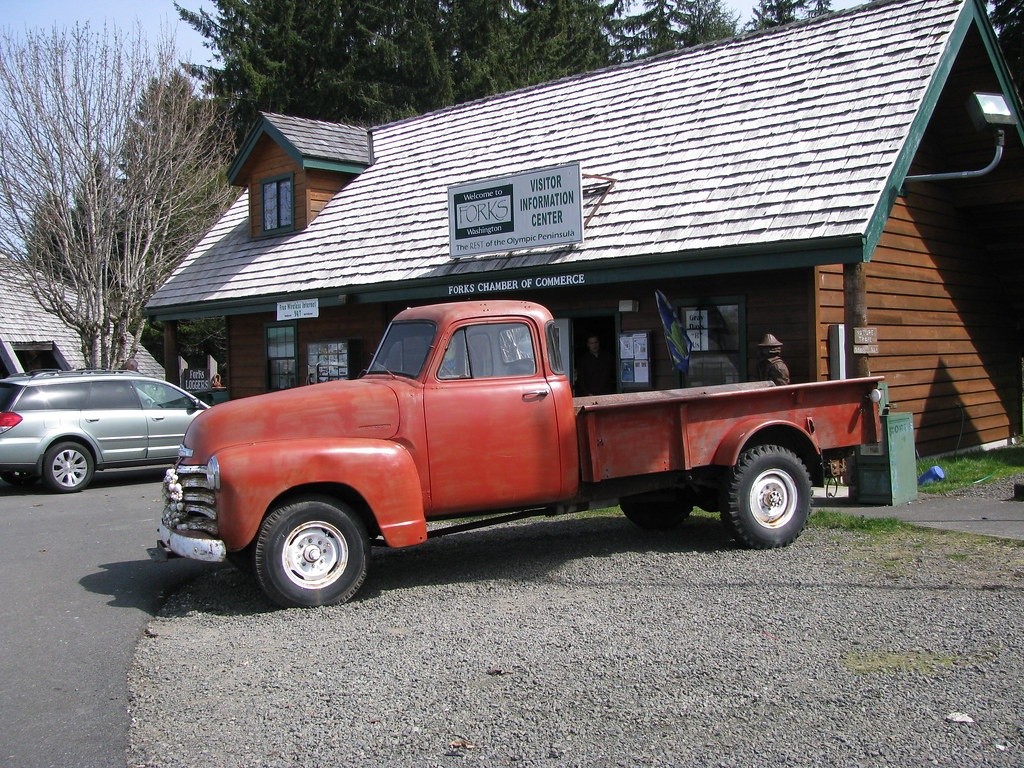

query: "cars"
xmin=0 ymin=369 xmax=215 ymax=491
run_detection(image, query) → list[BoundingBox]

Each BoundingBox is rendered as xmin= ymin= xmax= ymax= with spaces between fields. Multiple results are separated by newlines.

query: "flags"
xmin=655 ymin=290 xmax=692 ymax=375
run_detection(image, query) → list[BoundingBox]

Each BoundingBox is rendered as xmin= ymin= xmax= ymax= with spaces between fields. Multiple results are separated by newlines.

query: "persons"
xmin=756 ymin=334 xmax=789 ymax=386
xmin=579 ymin=335 xmax=616 ymax=395
xmin=127 ymin=360 xmax=138 ymax=371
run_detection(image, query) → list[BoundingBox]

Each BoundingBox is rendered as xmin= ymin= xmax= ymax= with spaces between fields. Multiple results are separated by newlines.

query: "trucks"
xmin=147 ymin=298 xmax=894 ymax=610
